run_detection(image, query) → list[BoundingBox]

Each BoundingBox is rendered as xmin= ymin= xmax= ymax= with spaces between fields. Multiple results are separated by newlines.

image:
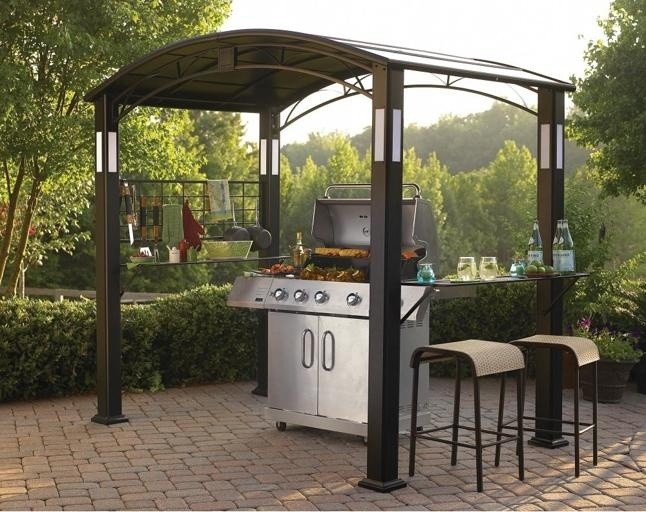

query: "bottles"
xmin=510 ymin=219 xmax=576 ymax=278
xmin=179 ymin=240 xmax=188 ymax=262
xmin=457 ymin=256 xmax=498 ymax=282
xmin=416 ymin=264 xmax=435 ymax=282
xmin=292 ymin=232 xmax=312 ymax=266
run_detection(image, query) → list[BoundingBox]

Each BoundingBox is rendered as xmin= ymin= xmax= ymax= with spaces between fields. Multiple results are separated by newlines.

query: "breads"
xmin=314 ymin=247 xmax=340 ymax=256
xmin=340 ymin=249 xmax=369 ymax=258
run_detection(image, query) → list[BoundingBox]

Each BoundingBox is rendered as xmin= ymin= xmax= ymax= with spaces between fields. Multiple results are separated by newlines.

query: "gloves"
xmin=160 ymin=203 xmax=206 ymax=252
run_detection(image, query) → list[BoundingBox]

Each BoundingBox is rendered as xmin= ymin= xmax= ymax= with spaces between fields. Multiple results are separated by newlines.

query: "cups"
xmin=166 ymin=249 xmax=197 ymax=263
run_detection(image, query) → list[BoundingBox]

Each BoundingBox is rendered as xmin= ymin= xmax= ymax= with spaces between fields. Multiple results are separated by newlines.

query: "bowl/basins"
xmin=203 ymin=241 xmax=253 ymax=259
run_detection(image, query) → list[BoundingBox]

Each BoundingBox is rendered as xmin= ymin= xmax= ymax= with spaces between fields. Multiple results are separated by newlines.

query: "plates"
xmin=130 ymin=256 xmax=154 ymax=264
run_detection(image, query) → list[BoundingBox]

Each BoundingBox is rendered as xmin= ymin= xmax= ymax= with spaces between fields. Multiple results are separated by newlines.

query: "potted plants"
xmin=582 ymin=328 xmax=646 ymax=405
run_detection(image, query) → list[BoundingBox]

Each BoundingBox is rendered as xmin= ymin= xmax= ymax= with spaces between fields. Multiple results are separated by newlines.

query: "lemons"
xmin=486 ymin=262 xmax=496 ymax=269
xmin=458 ymin=263 xmax=470 ymax=270
xmin=527 ymin=259 xmax=554 ymax=275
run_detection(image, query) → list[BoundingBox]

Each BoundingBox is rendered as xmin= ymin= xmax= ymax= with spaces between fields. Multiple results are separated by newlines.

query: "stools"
xmin=405 ymin=332 xmax=601 ymax=492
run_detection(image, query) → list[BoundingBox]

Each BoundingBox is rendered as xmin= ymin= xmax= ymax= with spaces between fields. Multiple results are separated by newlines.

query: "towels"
xmin=206 ymin=176 xmax=234 ymax=222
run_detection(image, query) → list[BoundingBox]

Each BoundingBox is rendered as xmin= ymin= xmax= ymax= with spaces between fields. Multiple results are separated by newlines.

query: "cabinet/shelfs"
xmin=256 ymin=316 xmax=429 ymax=437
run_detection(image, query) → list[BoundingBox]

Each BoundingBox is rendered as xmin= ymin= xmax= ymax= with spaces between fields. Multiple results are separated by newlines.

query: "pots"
xmin=223 ymin=199 xmax=272 ymax=252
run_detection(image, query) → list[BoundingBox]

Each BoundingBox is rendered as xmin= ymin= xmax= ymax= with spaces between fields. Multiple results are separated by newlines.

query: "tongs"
xmin=120 ymin=179 xmax=160 ymax=262
